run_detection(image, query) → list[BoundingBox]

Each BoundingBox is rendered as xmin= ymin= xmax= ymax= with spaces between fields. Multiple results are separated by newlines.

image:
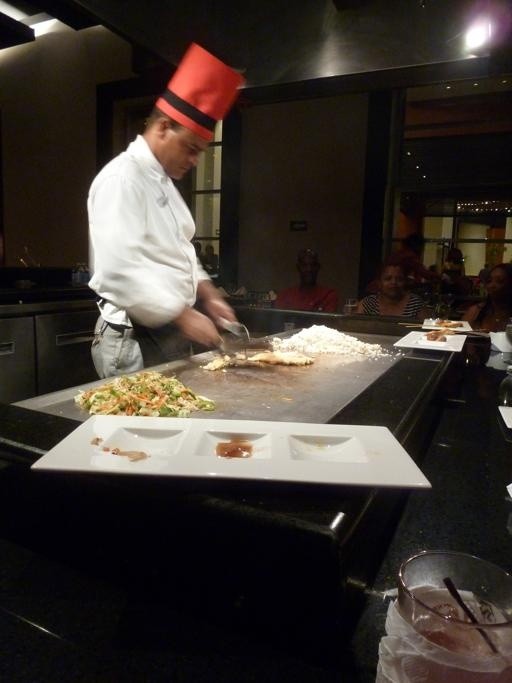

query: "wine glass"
xmin=506 ymin=318 xmax=512 ymax=374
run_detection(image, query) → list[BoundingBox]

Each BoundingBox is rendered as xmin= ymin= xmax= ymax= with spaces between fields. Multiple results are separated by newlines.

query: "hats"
xmin=154 ymin=41 xmax=247 ymax=142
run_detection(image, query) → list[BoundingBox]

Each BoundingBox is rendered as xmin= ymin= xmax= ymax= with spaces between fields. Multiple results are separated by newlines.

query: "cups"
xmin=247 ymin=292 xmax=271 ymax=310
xmin=344 ymin=298 xmax=359 ymax=315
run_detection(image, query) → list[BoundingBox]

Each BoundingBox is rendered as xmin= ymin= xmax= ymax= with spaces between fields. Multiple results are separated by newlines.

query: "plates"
xmin=393 ymin=318 xmax=474 ymax=352
xmin=28 ymin=414 xmax=433 ymax=489
xmin=499 ymin=403 xmax=512 ymax=429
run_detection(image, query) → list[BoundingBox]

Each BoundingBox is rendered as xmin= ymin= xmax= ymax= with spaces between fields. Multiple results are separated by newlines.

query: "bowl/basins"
xmin=395 ymin=551 xmax=512 ymax=661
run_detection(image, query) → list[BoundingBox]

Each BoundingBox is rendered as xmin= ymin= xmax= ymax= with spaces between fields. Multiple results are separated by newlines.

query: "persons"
xmin=87 ymin=105 xmax=239 ymax=382
xmin=354 ymin=247 xmax=512 ymax=330
xmin=270 ymin=249 xmax=339 ymax=315
